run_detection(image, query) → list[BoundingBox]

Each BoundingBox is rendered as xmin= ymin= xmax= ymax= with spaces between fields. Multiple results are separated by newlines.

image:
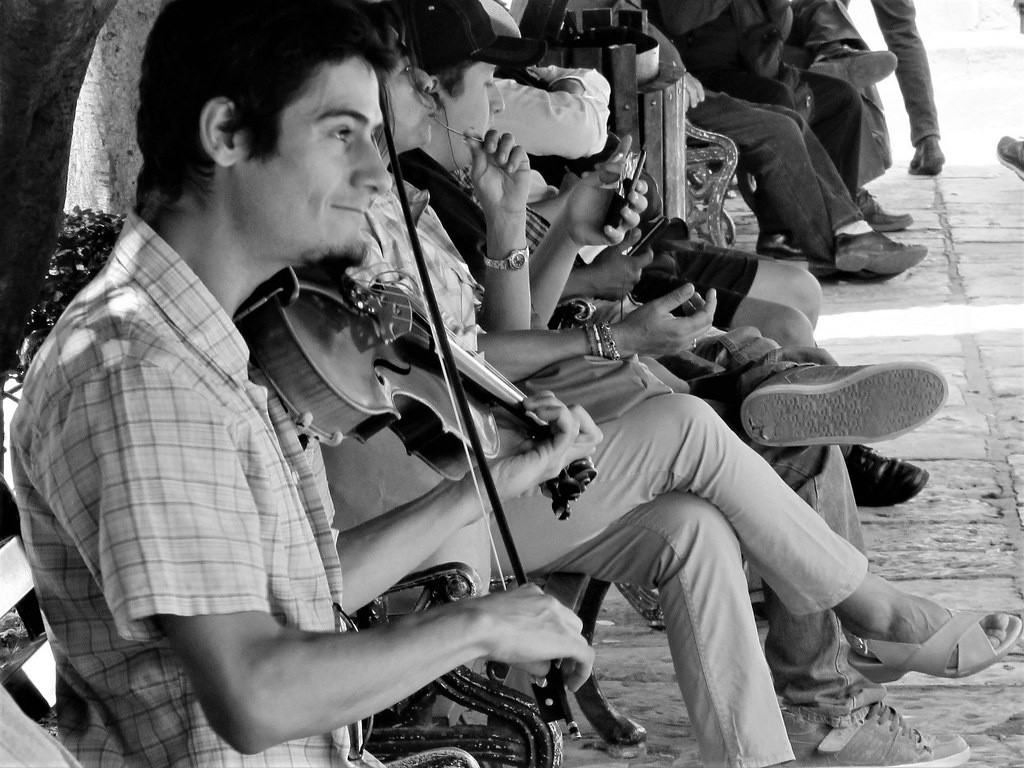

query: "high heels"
xmin=838 ymin=601 xmax=1024 ymax=684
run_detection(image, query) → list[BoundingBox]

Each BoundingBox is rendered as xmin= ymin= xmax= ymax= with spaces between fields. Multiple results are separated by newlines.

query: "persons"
xmin=346 ymin=0 xmax=1024 ymax=768
xmin=9 ymin=0 xmax=605 ymax=767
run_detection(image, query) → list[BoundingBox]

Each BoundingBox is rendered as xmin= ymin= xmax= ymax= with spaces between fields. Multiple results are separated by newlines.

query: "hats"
xmin=397 ymin=0 xmax=551 ymax=74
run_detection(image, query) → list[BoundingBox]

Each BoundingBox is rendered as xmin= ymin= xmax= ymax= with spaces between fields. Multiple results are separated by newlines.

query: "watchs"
xmin=476 ymin=237 xmax=530 ymax=271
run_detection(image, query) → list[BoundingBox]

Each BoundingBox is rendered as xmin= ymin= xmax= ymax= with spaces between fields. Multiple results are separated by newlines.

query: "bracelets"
xmin=584 ymin=322 xmax=621 ymax=361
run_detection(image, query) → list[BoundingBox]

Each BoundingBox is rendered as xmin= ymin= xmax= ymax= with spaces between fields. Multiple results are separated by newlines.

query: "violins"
xmin=230 ymin=264 xmax=597 ymax=520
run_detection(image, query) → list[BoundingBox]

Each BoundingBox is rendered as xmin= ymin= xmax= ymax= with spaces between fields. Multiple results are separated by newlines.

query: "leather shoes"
xmin=844 ymin=446 xmax=930 ymax=508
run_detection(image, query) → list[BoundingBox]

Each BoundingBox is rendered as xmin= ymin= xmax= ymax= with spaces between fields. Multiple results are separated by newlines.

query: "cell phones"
xmin=629 ymin=217 xmax=667 ymax=257
xmin=603 ymin=153 xmax=646 ymax=230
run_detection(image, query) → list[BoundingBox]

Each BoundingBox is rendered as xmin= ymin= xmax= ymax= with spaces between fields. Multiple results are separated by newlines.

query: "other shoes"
xmin=753 ymin=233 xmax=807 ymax=263
xmin=741 ymin=355 xmax=952 ymax=449
xmin=997 ymin=135 xmax=1024 ymax=179
xmin=907 ymin=136 xmax=946 ymax=177
xmin=853 ymin=190 xmax=916 ymax=232
xmin=773 ymin=700 xmax=974 ymax=768
xmin=808 ymin=46 xmax=900 ymax=91
xmin=835 ymin=227 xmax=929 ymax=275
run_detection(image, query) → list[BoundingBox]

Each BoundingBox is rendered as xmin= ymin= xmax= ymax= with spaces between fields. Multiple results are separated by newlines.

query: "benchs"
xmin=0 ymin=117 xmax=742 ymax=768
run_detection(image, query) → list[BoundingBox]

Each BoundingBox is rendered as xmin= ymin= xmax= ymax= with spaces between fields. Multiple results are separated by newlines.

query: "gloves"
xmin=528 ymin=64 xmax=612 ymax=104
